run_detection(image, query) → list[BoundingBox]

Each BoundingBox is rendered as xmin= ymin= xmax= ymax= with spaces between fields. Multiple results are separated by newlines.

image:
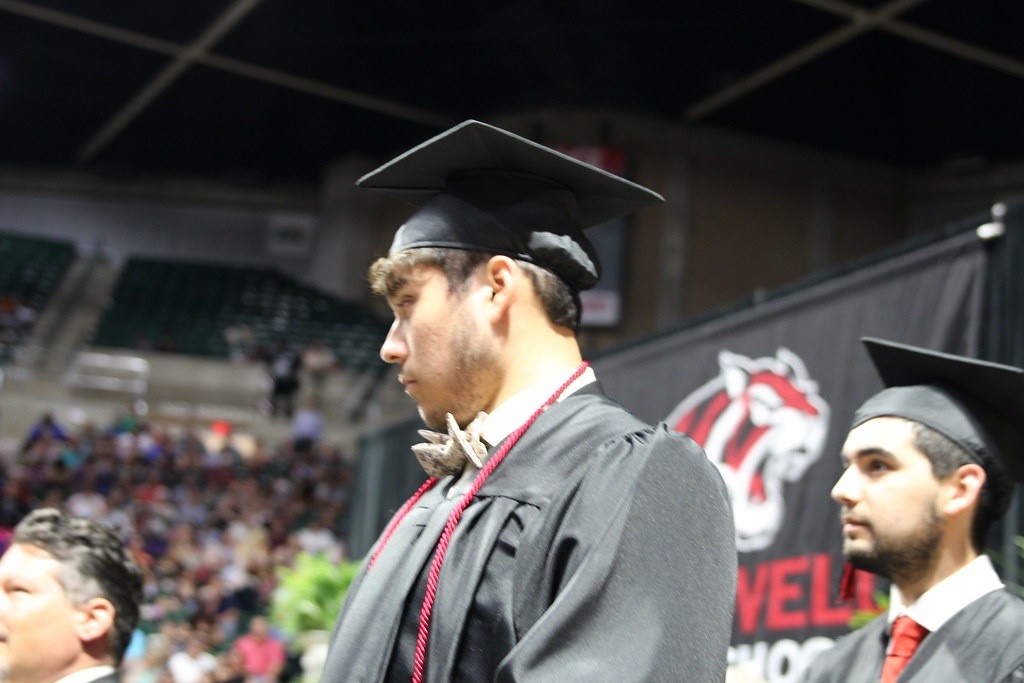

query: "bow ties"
xmin=410 ymin=410 xmax=487 ymax=481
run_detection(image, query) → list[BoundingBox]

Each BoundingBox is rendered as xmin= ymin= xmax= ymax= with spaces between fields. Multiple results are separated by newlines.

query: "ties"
xmin=880 ymin=617 xmax=928 ymax=683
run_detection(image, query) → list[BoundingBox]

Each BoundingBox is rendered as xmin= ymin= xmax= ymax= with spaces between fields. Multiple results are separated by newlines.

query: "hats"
xmin=356 ymin=120 xmax=666 ymax=292
xmin=847 ymin=336 xmax=1024 ymax=521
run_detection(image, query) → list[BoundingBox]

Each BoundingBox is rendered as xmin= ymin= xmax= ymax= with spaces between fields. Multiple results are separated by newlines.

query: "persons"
xmin=794 ymin=336 xmax=1024 ymax=683
xmin=0 ymin=507 xmax=144 ymax=683
xmin=0 ymin=413 xmax=358 ymax=683
xmin=249 ymin=319 xmax=339 ymax=420
xmin=319 ymin=118 xmax=738 ymax=683
